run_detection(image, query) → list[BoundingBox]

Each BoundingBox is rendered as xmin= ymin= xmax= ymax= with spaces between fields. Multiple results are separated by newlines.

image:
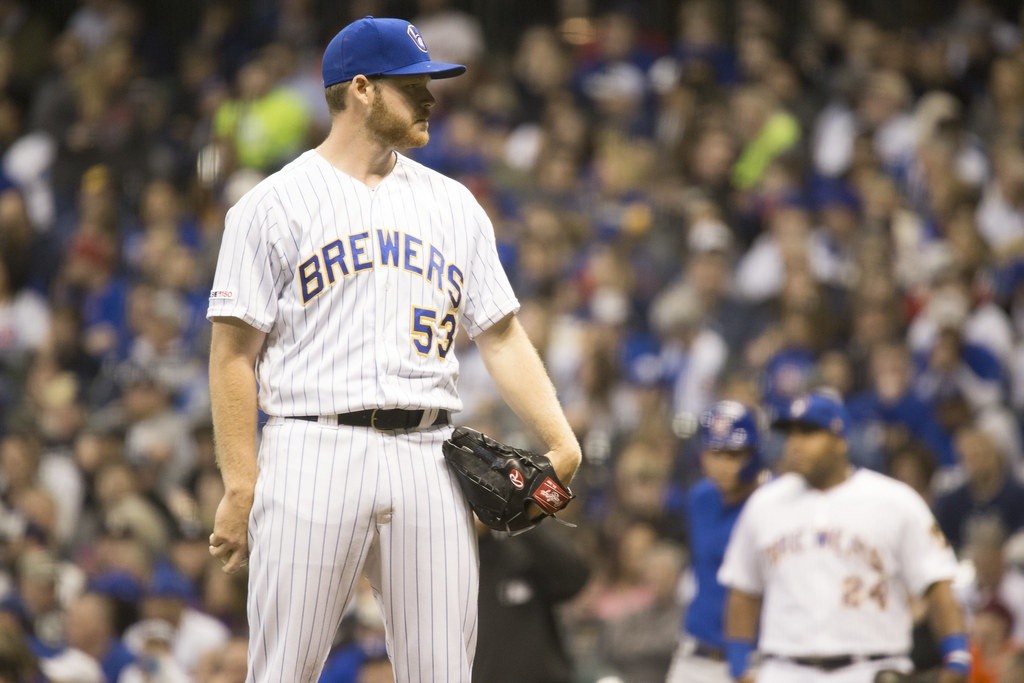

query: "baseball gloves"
xmin=442 ymin=424 xmax=574 ymax=539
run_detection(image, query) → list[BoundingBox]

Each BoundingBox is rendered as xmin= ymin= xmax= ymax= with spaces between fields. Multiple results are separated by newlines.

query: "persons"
xmin=718 ymin=393 xmax=975 ymax=683
xmin=0 ymin=1 xmax=1024 ymax=683
xmin=208 ymin=16 xmax=581 ymax=683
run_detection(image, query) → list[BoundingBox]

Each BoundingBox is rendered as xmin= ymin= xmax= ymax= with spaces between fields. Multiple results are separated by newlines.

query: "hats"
xmin=769 ymin=386 xmax=848 ymax=436
xmin=322 ymin=15 xmax=467 ymax=91
xmin=697 ymin=400 xmax=760 ymax=451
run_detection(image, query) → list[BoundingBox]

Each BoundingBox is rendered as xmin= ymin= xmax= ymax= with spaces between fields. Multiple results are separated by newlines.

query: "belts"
xmin=789 ymin=653 xmax=887 ymax=671
xmin=694 ymin=642 xmax=729 ymax=661
xmin=285 ymin=407 xmax=449 ymax=432
